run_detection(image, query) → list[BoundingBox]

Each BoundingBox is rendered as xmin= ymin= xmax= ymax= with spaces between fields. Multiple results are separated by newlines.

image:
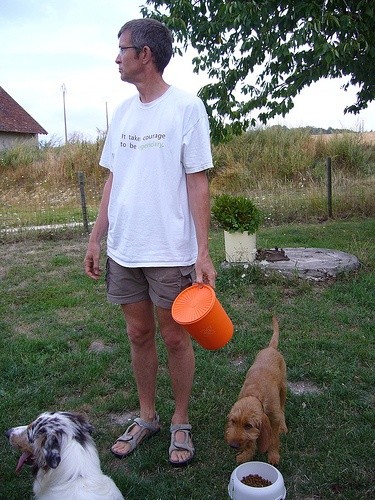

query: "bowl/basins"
xmin=227 ymin=461 xmax=286 ymax=500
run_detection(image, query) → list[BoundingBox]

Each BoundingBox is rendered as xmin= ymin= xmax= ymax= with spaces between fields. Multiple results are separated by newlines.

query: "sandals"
xmin=111 ymin=412 xmax=162 ymax=457
xmin=169 ymin=422 xmax=196 ymax=466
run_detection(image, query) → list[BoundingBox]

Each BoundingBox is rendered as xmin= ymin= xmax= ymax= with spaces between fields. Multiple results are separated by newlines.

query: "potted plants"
xmin=210 ymin=194 xmax=262 ymax=263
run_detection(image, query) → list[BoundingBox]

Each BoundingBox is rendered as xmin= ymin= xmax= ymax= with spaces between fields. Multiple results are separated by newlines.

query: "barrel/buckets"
xmin=172 ymin=284 xmax=234 ymax=350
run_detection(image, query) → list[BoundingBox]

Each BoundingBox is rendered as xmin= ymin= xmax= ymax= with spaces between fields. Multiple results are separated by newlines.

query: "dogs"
xmin=7 ymin=411 xmax=124 ymax=500
xmin=224 ymin=317 xmax=288 ymax=465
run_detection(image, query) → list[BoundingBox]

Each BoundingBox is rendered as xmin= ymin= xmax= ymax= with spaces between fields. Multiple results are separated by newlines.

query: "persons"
xmin=83 ymin=19 xmax=214 ymax=465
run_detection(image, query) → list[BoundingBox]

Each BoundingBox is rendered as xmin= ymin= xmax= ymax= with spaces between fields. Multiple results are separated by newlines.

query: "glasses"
xmin=119 ymin=46 xmax=142 ymax=52
